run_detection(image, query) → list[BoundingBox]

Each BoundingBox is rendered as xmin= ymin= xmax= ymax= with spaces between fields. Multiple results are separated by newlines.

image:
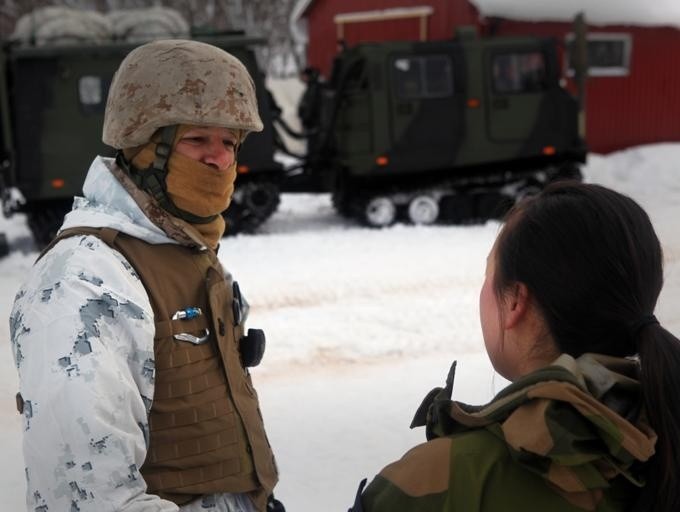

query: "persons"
xmin=347 ymin=179 xmax=680 ymax=510
xmin=9 ymin=37 xmax=290 ymax=512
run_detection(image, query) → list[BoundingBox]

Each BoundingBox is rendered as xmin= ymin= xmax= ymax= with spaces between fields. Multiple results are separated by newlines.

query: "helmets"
xmin=102 ymin=40 xmax=264 ymax=149
